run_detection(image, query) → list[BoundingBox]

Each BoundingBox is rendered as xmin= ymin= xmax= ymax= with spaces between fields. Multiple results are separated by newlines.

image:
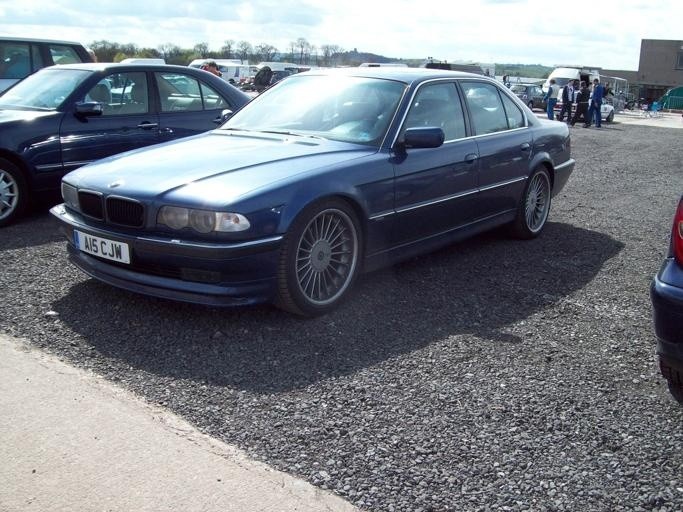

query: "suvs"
xmin=0 ymin=36 xmax=97 ymax=95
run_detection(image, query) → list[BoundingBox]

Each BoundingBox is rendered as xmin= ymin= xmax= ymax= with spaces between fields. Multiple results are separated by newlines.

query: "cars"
xmin=553 ymin=87 xmax=615 ymax=122
xmin=511 ymin=83 xmax=547 ymax=112
xmin=267 ymin=69 xmax=291 ymax=86
xmin=49 ymin=67 xmax=576 ymax=318
xmin=0 ymin=61 xmax=253 ymax=228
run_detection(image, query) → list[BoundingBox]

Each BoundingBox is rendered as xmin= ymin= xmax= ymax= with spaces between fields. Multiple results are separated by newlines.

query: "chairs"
xmin=86 ymin=82 xmax=201 ymax=114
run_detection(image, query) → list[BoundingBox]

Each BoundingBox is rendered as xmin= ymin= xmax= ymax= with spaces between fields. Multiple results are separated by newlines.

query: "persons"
xmin=601 ymin=82 xmax=614 ymax=103
xmin=581 ymin=78 xmax=601 ymax=129
xmin=204 ymin=61 xmax=223 ymax=79
xmin=557 ymin=78 xmax=575 ymax=121
xmin=568 ymin=81 xmax=591 ymax=128
xmin=541 ymin=80 xmax=559 ymax=120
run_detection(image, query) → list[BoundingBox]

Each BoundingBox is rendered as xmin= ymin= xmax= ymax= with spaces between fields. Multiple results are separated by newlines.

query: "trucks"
xmin=541 ymin=67 xmax=628 ymax=114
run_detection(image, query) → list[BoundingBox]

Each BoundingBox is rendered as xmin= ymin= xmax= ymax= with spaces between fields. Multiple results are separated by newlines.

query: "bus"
xmin=190 ymin=59 xmax=240 ymax=84
